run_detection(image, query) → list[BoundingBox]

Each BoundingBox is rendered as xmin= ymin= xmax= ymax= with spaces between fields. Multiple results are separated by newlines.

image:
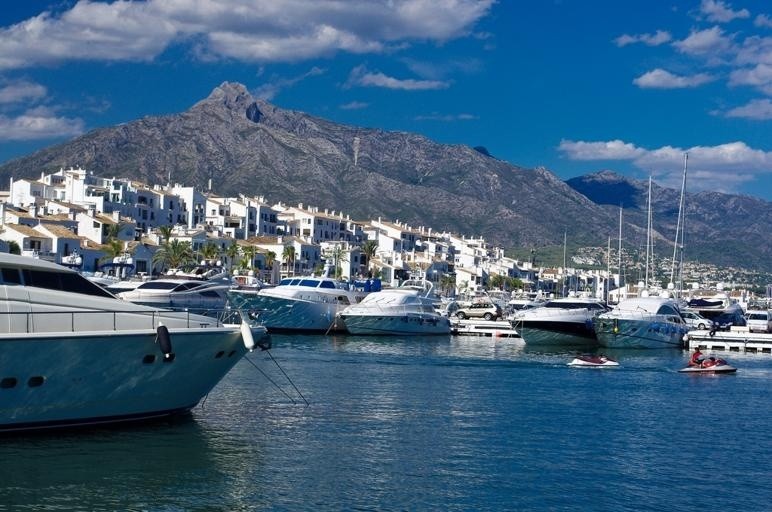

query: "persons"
xmin=701 ymin=356 xmax=716 ymax=368
xmin=691 ymin=347 xmax=703 ymax=368
